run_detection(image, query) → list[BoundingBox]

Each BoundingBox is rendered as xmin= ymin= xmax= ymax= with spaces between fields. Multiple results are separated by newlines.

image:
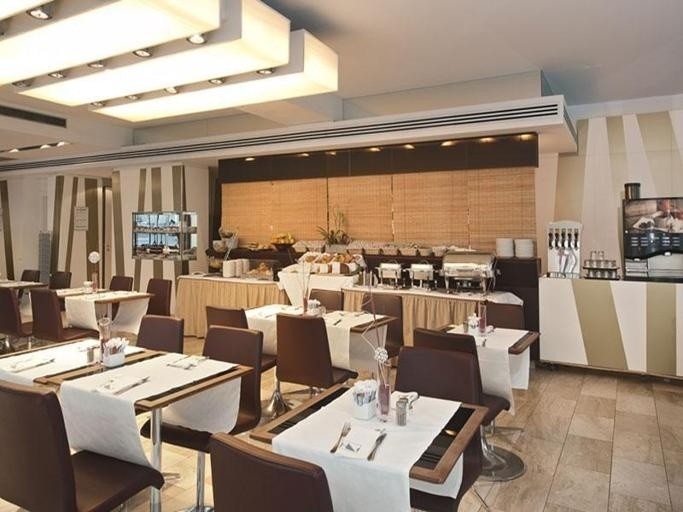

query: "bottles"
xmin=92 ymin=345 xmax=100 ymax=361
xmin=395 ymin=400 xmax=406 ymax=425
xmin=399 ymin=397 xmax=409 ymax=420
xmin=463 ymin=320 xmax=468 ymax=333
xmin=87 ymin=346 xmax=93 ymax=362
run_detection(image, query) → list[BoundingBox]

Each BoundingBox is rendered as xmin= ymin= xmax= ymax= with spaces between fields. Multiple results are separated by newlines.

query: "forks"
xmin=329 ymin=421 xmax=352 ymax=453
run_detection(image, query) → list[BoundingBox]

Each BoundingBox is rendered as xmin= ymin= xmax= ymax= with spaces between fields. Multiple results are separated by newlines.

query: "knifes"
xmin=367 ymin=433 xmax=388 ymax=462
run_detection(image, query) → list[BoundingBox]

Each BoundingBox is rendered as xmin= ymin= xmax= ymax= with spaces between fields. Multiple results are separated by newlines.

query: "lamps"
xmin=0 ymin=5 xmax=275 ymax=108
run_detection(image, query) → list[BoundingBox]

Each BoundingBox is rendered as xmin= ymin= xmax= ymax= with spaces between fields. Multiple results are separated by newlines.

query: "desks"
xmin=537 ymin=274 xmax=683 ymax=383
xmin=176 ymin=274 xmax=526 ymax=369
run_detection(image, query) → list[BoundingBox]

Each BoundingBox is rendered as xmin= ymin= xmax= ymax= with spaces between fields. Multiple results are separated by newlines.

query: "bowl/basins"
xmin=213 ymin=240 xmax=225 ymax=252
xmin=417 ymin=248 xmax=432 ymax=257
xmin=223 ymin=259 xmax=249 ymax=278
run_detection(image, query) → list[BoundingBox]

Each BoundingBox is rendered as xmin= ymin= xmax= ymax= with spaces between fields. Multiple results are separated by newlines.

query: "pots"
xmin=624 ymin=182 xmax=641 ymax=199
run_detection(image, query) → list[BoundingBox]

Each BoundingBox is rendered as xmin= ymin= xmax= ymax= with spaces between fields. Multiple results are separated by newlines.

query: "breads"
xmin=305 ymin=255 xmax=315 ymax=262
xmin=315 ymin=255 xmax=332 ymax=264
xmin=247 ymin=263 xmax=274 ymax=276
xmin=333 ymin=254 xmax=359 ymax=265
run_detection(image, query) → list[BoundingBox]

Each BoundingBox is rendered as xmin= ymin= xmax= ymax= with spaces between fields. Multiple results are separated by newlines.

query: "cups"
xmin=584 ymin=250 xmax=617 ymax=279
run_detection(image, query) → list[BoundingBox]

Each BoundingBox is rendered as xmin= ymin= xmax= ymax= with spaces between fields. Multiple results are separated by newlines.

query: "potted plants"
xmin=316 ymin=214 xmax=349 ymax=253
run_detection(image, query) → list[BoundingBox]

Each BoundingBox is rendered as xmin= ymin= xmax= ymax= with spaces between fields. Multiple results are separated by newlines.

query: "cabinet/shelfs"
xmin=130 ymin=211 xmax=196 ymax=263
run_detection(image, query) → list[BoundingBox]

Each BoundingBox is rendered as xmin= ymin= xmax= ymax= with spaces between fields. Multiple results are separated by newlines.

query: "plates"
xmin=222 ymin=237 xmax=239 ymax=250
xmin=496 ymin=238 xmax=534 ymax=260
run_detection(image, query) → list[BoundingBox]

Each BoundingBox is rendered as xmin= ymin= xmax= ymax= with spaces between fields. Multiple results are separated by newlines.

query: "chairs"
xmin=0 ymin=383 xmax=165 ymax=512
xmin=208 ymin=431 xmax=333 ymax=512
xmin=478 ymin=300 xmax=524 ymax=330
xmin=310 ymin=288 xmax=344 ymax=313
xmin=204 ymin=304 xmax=279 ymax=373
xmin=394 ymin=345 xmax=481 ymax=410
xmin=135 ymin=324 xmax=265 ymax=511
xmin=361 ymin=291 xmax=404 ymax=359
xmin=0 ymin=268 xmax=187 ymax=353
xmin=272 ymin=312 xmax=353 ymax=401
xmin=412 ymin=326 xmax=530 ymax=481
